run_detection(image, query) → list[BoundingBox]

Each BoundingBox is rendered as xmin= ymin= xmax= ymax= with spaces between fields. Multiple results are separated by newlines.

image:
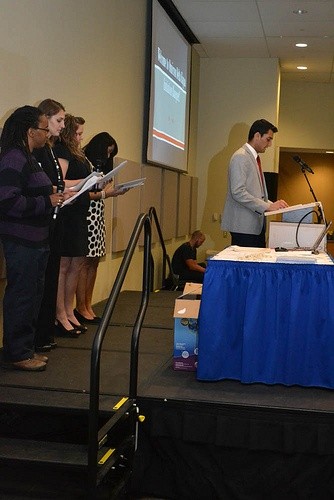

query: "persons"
xmin=171 ymin=231 xmax=206 ymax=284
xmin=0 ymin=98 xmax=130 ymax=371
xmin=221 ymin=119 xmax=289 ymax=248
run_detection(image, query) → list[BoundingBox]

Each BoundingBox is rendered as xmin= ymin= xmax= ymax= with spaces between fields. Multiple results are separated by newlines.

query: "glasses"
xmin=27 ymin=126 xmax=49 ymax=134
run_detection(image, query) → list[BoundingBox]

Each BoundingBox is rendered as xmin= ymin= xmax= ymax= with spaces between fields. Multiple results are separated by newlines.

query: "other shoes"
xmin=2 ymin=358 xmax=47 ymax=372
xmin=74 ymin=307 xmax=100 ymax=324
xmin=34 ymin=341 xmax=56 ymax=351
xmin=33 ymin=353 xmax=48 ymax=361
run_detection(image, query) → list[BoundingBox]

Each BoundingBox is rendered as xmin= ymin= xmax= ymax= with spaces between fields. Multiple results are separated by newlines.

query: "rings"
xmin=57 ymin=203 xmax=60 ymax=206
xmin=58 ymin=199 xmax=61 ymax=202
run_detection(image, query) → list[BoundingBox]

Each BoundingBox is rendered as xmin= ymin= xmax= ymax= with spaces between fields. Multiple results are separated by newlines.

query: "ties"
xmin=257 ymin=156 xmax=264 ymax=187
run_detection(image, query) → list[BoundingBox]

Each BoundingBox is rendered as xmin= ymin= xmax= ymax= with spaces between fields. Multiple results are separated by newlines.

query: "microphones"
xmin=293 ymin=156 xmax=314 ymax=174
xmin=52 ymin=181 xmax=65 ymax=219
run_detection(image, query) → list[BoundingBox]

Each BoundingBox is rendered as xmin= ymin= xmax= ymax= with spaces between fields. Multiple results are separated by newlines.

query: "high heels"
xmin=54 ymin=318 xmax=81 ymax=338
xmin=68 ymin=317 xmax=88 ymax=331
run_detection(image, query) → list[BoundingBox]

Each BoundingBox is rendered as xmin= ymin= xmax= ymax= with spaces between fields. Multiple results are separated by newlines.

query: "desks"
xmin=197 ymin=246 xmax=334 ymax=390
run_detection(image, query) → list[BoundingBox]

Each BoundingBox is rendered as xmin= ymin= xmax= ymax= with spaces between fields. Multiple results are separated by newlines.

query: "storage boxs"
xmin=173 ymin=282 xmax=203 ymax=372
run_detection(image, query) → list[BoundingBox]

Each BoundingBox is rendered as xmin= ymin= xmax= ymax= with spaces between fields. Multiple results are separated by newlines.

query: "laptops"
xmin=287 ymin=221 xmax=332 ymax=254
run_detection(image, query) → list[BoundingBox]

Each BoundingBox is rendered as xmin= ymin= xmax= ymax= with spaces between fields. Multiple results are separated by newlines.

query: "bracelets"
xmin=101 ymin=191 xmax=106 ymax=199
